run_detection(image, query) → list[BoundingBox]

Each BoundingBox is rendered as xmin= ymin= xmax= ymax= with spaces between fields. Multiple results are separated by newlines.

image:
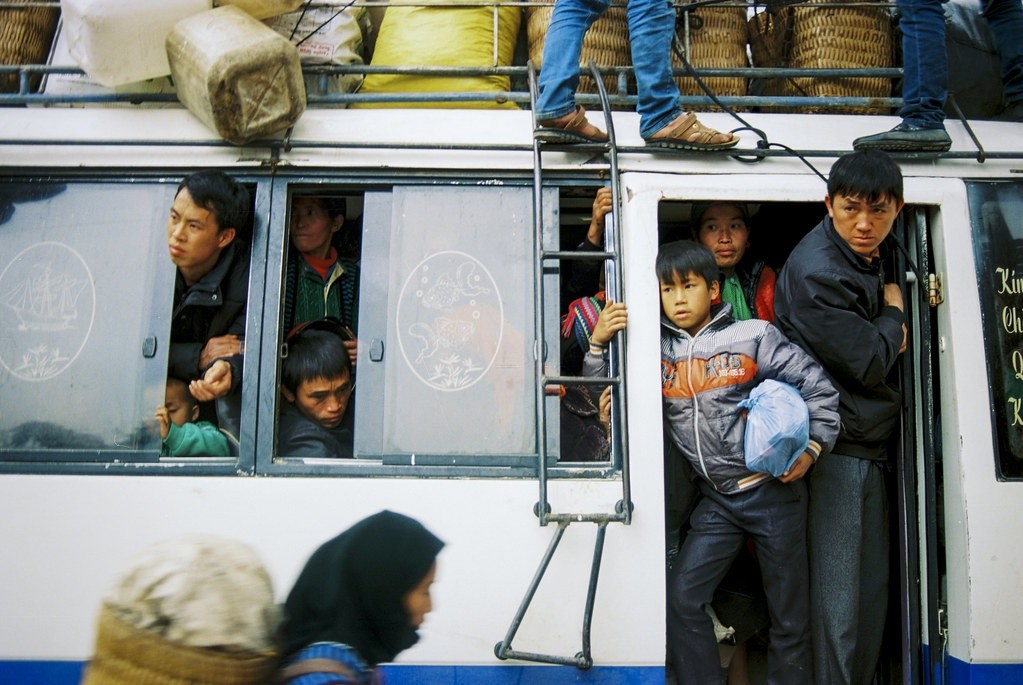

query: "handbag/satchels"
xmin=736 ymin=378 xmax=809 ymax=478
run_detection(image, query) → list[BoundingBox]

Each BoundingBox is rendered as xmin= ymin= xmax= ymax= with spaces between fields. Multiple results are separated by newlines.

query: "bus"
xmin=0 ymin=62 xmax=1023 ymax=685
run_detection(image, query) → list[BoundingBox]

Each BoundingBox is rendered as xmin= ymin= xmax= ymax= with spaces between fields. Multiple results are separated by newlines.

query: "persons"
xmin=144 ymin=169 xmax=370 ymax=460
xmin=535 ymin=0 xmax=740 ymax=150
xmin=276 ymin=510 xmax=445 ymax=685
xmin=853 ymin=0 xmax=952 ymax=153
xmin=561 ymin=151 xmax=907 ymax=685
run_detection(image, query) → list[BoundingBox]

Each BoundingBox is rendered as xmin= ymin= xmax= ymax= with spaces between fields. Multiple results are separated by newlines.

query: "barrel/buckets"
xmin=58 ymin=1 xmax=307 ymax=145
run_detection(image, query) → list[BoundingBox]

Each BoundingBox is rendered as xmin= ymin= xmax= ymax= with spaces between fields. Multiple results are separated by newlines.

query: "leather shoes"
xmin=852 ymin=122 xmax=952 ymax=152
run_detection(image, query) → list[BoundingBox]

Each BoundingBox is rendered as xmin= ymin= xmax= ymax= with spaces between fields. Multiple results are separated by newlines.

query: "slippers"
xmin=644 ymin=110 xmax=740 ymax=151
xmin=533 ymin=104 xmax=611 ymax=143
